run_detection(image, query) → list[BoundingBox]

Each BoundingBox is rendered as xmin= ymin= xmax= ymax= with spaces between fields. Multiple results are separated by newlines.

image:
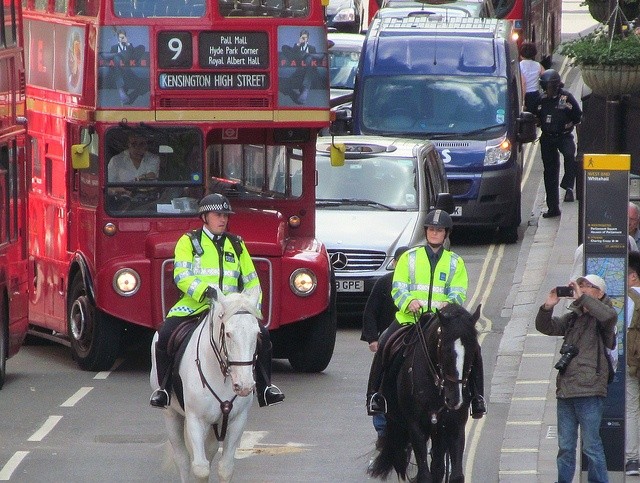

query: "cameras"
xmin=553 ymin=342 xmax=580 ymax=374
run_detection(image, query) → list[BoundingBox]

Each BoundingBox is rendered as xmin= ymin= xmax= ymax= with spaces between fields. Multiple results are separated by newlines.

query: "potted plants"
xmin=557 ymin=28 xmax=640 ymax=98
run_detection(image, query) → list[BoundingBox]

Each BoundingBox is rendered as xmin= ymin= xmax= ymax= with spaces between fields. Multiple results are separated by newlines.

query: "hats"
xmin=574 ymin=274 xmax=607 ymax=293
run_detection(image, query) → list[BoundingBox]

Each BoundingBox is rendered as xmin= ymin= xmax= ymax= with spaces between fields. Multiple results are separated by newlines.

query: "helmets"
xmin=539 ymin=69 xmax=560 ymax=84
xmin=424 ymin=210 xmax=452 ymax=228
xmin=197 ymin=194 xmax=235 ymax=215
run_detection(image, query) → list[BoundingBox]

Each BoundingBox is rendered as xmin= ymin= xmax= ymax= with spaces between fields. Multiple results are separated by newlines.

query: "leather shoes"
xmin=564 ymin=188 xmax=574 ymax=201
xmin=543 ymin=211 xmax=560 ymax=218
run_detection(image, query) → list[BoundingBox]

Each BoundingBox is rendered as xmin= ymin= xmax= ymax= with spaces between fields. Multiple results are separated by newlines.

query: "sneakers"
xmin=625 ymin=458 xmax=638 ymax=474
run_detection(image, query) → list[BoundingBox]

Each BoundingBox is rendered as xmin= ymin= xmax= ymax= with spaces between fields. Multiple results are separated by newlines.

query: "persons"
xmin=366 ymin=209 xmax=486 ymax=418
xmin=565 ymin=235 xmax=638 ymax=309
xmin=535 ymin=69 xmax=582 ymax=218
xmin=519 ymin=42 xmax=546 ymax=112
xmin=149 ymin=193 xmax=285 ymax=408
xmin=625 ymin=200 xmax=639 ymax=252
xmin=107 ymin=132 xmax=161 ymax=212
xmin=361 ymin=246 xmax=412 ymax=451
xmin=281 ymin=30 xmax=316 ymax=104
xmin=109 ymin=29 xmax=145 ymax=105
xmin=535 ymin=274 xmax=618 ymax=483
xmin=625 ymin=255 xmax=640 ymax=476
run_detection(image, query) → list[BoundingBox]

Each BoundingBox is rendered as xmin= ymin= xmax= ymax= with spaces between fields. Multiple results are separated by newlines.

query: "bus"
xmin=367 ymin=0 xmax=561 ymax=70
xmin=0 ymin=19 xmax=35 ymax=387
xmin=26 ymin=0 xmax=337 ymax=375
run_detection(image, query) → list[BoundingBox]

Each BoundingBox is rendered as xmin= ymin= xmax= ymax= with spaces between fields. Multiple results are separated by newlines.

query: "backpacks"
xmin=594 ymin=319 xmax=618 ymax=384
xmin=626 ymin=288 xmax=640 ymax=377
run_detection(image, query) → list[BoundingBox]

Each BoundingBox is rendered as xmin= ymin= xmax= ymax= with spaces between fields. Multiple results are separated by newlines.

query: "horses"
xmin=150 ymin=285 xmax=261 ymax=483
xmin=358 ymin=303 xmax=485 ymax=483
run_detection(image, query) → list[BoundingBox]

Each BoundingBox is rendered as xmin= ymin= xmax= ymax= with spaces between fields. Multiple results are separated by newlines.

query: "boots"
xmin=150 ymin=342 xmax=171 ymax=406
xmin=472 ymin=364 xmax=485 ymax=419
xmin=256 ymin=342 xmax=285 ymax=407
xmin=366 ymin=353 xmax=385 ymax=415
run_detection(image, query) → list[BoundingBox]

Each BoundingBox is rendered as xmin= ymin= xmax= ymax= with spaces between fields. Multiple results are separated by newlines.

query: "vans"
xmin=351 ymin=16 xmax=537 ymax=242
xmin=268 ymin=135 xmax=456 ymax=309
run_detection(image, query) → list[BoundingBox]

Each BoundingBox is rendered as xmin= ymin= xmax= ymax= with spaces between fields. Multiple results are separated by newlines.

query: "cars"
xmin=326 ymin=1 xmax=365 ymax=33
xmin=327 ymin=32 xmax=366 ymax=135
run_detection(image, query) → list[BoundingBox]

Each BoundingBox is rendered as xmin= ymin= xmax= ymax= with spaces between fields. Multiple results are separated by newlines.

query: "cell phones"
xmin=556 ymin=286 xmax=574 ymax=296
